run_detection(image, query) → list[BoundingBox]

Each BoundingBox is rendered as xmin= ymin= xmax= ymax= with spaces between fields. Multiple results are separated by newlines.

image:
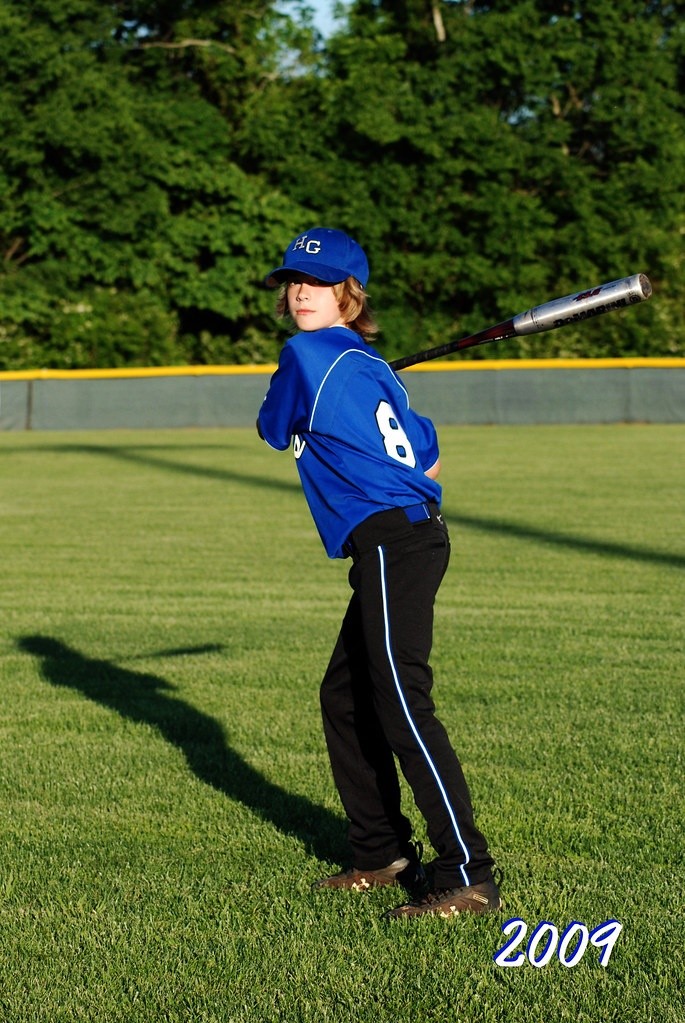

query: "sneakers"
xmin=312 ymin=841 xmax=425 ymax=890
xmin=381 ymin=876 xmax=502 ymax=919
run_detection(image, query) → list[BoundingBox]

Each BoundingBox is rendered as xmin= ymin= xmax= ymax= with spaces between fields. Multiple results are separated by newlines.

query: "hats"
xmin=266 ymin=228 xmax=369 ymax=287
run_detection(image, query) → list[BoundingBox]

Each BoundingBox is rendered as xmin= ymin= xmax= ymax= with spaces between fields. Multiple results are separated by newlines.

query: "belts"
xmin=347 ymin=503 xmax=431 ymax=555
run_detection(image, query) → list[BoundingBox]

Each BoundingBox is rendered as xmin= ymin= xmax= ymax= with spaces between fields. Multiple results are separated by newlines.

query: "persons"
xmin=257 ymin=229 xmax=503 ymax=917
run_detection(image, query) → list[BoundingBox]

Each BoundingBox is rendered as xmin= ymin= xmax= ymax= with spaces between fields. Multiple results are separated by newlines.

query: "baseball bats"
xmin=384 ymin=271 xmax=654 ymax=371
